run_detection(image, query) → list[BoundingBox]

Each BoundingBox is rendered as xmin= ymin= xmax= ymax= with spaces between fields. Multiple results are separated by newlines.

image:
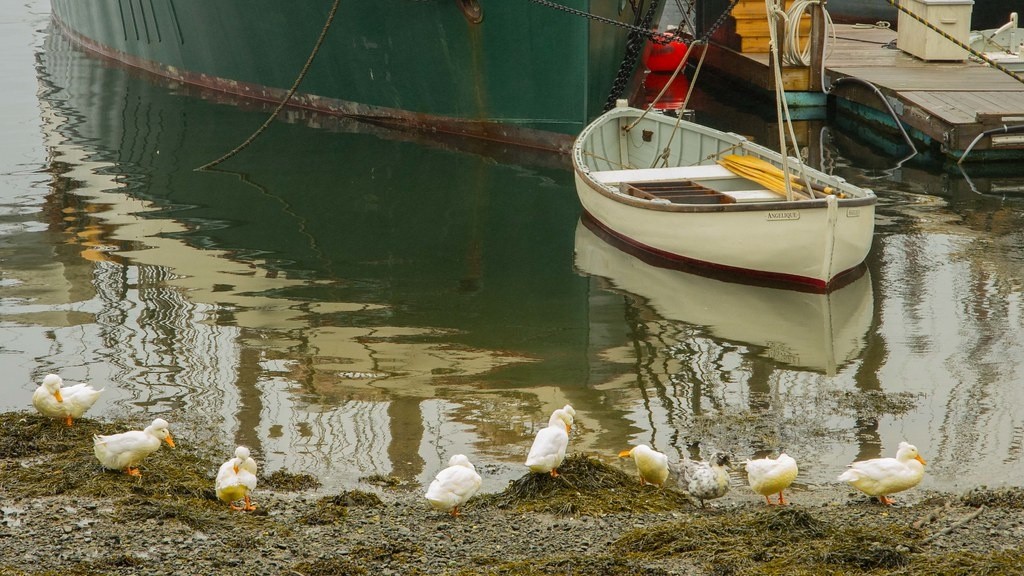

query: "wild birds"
xmin=523 ymin=403 xmax=576 ymax=478
xmin=32 ymin=373 xmax=105 ymax=426
xmin=618 ymin=443 xmax=669 ymax=489
xmin=679 ymin=451 xmax=733 ymax=510
xmin=93 ymin=417 xmax=175 ymax=478
xmin=746 ymin=453 xmax=799 ymax=505
xmin=424 ymin=455 xmax=482 ymax=516
xmin=214 ymin=445 xmax=258 ymax=512
xmin=837 ymin=440 xmax=927 ymax=505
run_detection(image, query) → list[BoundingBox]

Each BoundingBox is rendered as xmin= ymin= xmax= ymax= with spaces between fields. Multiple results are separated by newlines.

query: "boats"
xmin=572 ymin=99 xmax=879 ymax=290
xmin=50 ymin=1 xmax=667 ymax=156
xmin=573 ymin=212 xmax=881 ymax=379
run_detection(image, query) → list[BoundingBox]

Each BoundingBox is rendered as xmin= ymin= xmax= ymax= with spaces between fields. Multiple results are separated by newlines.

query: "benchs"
xmin=592 ymin=165 xmax=765 ymax=192
xmin=719 ymin=190 xmax=789 ymax=202
